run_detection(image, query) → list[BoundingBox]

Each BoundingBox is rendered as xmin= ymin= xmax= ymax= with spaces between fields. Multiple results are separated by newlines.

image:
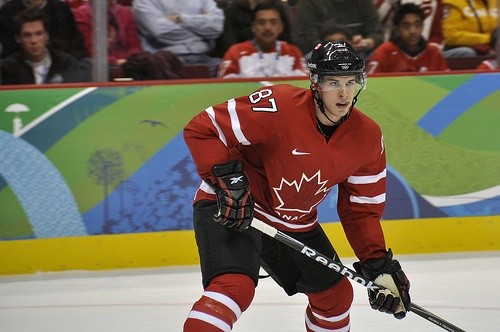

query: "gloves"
xmin=353 ymin=247 xmax=412 ymax=319
xmin=203 ymin=158 xmax=255 ymax=233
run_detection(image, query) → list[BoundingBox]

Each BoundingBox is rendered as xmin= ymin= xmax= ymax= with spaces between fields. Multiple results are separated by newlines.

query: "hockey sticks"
xmin=218 ymin=208 xmax=466 ymax=332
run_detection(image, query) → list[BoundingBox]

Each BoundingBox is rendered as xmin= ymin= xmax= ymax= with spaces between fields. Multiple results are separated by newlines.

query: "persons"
xmin=0 ymin=0 xmax=499 ymax=86
xmin=182 ymin=40 xmax=411 ymax=332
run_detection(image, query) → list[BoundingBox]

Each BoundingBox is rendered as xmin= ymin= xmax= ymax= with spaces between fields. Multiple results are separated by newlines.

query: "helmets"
xmin=306 ymin=38 xmax=365 ymax=75
